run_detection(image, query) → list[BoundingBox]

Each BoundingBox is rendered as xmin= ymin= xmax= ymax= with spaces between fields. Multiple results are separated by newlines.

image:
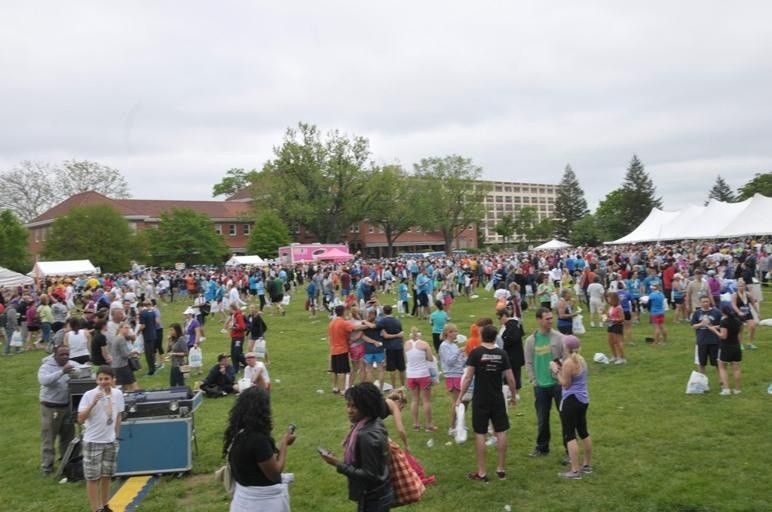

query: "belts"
xmin=40 ymin=400 xmax=71 ymax=409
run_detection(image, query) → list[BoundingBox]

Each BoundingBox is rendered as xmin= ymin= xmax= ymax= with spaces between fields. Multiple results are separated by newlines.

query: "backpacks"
xmin=494 ymin=289 xmax=508 ymax=311
xmin=0 ymin=308 xmax=12 ymax=327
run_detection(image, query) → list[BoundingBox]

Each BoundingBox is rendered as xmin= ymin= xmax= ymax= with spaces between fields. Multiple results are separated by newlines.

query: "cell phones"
xmin=317 ymin=445 xmax=334 ymax=459
xmin=288 ymin=424 xmax=296 ymax=435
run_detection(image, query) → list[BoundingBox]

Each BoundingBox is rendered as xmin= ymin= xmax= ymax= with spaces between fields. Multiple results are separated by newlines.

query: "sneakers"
xmin=208 ymin=308 xmax=288 ymax=335
xmin=468 ymin=433 xmax=510 ymax=483
xmin=2 ymin=342 xmax=44 ymax=358
xmin=588 ymin=316 xmax=759 ymax=397
xmin=329 ymin=385 xmax=348 ymax=397
xmin=94 ymin=504 xmax=114 ymax=512
xmin=557 ymin=469 xmax=583 ymax=480
xmin=528 ymin=448 xmax=549 ymax=458
xmin=145 ymin=363 xmax=167 ymax=377
xmin=576 ymin=463 xmax=593 ymax=474
xmin=411 ymin=424 xmax=420 ymax=432
xmin=425 ymin=424 xmax=439 ymax=432
xmin=560 ymin=451 xmax=577 ymax=466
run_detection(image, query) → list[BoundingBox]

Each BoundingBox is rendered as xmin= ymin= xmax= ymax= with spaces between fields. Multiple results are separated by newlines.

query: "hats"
xmin=183 ymin=308 xmax=194 ymax=315
xmin=218 ymin=352 xmax=232 ymax=362
xmin=562 ymin=335 xmax=580 ymax=349
xmin=244 ymin=352 xmax=257 ymax=359
xmin=672 ymin=268 xmax=718 ymax=280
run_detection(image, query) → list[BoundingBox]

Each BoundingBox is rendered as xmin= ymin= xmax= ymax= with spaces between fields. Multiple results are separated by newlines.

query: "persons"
xmin=1 ymin=233 xmax=772 ymax=512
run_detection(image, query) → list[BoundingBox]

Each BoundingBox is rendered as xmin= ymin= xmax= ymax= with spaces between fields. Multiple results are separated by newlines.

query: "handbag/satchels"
xmin=452 ymin=402 xmax=468 ymax=444
xmin=550 ymin=293 xmax=559 ymax=310
xmin=188 ymin=346 xmax=203 ymax=368
xmin=176 ymin=360 xmax=193 ymax=374
xmin=386 ymin=436 xmax=438 ymax=508
xmin=304 ymin=296 xmax=311 ymax=311
xmin=214 ymin=429 xmax=244 ymax=496
xmin=127 ymin=354 xmax=142 ymax=371
xmin=9 ymin=330 xmax=23 ymax=347
xmin=280 ymin=295 xmax=291 ymax=306
xmin=568 ymin=304 xmax=586 ymax=334
xmin=424 ymin=354 xmax=442 ymax=387
xmin=396 ymin=300 xmax=408 ymax=315
xmin=252 ymin=337 xmax=266 ymax=359
xmin=436 ymin=290 xmax=446 ymax=305
